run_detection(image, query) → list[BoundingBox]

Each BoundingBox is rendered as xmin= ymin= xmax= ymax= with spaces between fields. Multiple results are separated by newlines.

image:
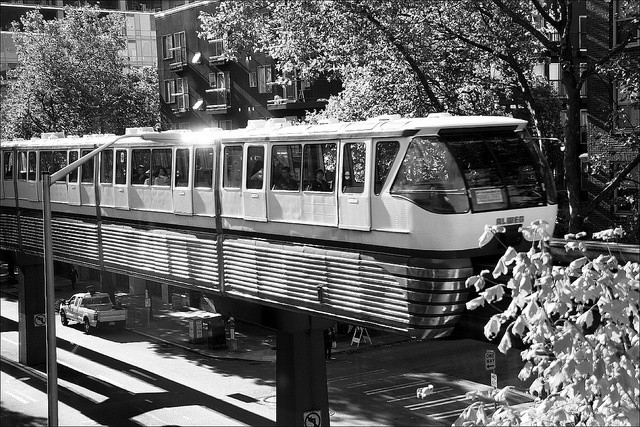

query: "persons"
xmin=271 ymin=166 xmax=300 ymax=191
xmin=68 ymin=265 xmax=79 ymax=291
xmin=154 ymin=167 xmax=170 ymax=186
xmin=323 ymin=326 xmax=337 ymax=361
xmin=143 ymin=169 xmax=156 ymax=185
xmin=137 ymin=165 xmax=147 ymax=184
xmin=304 ymin=168 xmax=333 ymax=191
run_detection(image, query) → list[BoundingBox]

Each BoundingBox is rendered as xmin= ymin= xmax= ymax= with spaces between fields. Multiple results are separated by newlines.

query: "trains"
xmin=0 ymin=112 xmax=558 ymax=339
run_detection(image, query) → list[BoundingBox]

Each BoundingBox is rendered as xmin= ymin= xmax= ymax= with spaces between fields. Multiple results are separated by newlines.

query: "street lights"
xmin=41 ymin=130 xmax=192 ymax=427
